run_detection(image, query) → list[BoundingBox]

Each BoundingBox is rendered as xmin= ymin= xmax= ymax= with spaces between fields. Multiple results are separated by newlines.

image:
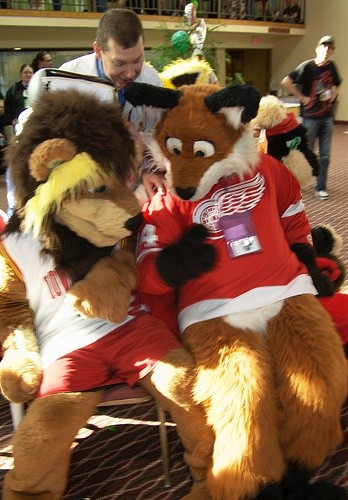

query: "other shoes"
xmin=310 ymin=165 xmax=320 ymax=187
xmin=314 ymin=190 xmax=329 ymax=200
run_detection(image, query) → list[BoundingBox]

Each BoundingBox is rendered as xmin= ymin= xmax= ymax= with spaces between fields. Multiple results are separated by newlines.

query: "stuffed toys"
xmin=0 ymin=59 xmax=348 ymax=500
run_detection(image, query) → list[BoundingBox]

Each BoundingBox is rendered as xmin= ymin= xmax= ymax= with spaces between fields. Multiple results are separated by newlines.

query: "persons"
xmin=45 ymin=8 xmax=167 ymax=201
xmin=0 ymin=49 xmax=54 ymax=222
xmin=0 ymin=0 xmax=305 ymax=24
xmin=281 ymin=35 xmax=343 ymax=201
xmin=188 ymin=46 xmax=219 ymax=87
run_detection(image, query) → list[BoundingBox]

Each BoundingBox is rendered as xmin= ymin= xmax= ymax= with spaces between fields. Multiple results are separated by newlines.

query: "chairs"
xmin=10 ymin=386 xmax=172 ymax=489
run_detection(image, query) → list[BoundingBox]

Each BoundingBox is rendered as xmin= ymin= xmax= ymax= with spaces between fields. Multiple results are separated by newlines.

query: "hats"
xmin=317 ymin=35 xmax=335 ymax=48
